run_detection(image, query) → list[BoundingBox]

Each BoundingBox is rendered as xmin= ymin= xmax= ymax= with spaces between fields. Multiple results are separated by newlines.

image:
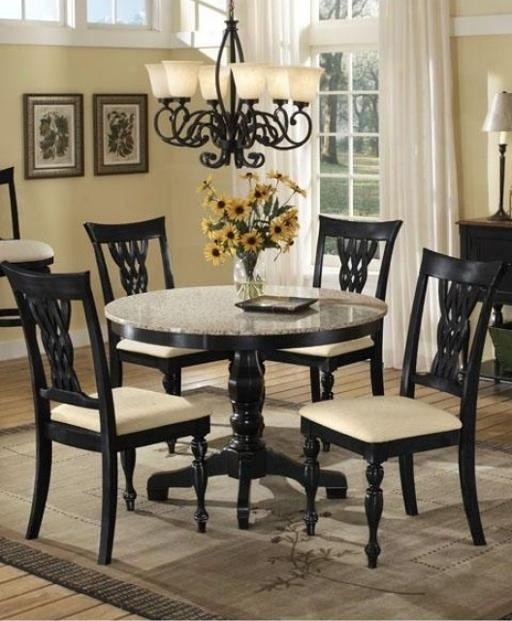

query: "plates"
xmin=235 ymin=295 xmax=315 ymax=311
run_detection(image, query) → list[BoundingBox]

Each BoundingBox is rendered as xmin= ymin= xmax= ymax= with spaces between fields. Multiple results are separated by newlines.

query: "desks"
xmin=104 ymin=284 xmax=390 ymax=531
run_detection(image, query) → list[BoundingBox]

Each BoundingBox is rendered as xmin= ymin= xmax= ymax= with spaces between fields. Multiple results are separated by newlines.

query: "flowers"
xmin=196 ymin=169 xmax=307 ymax=272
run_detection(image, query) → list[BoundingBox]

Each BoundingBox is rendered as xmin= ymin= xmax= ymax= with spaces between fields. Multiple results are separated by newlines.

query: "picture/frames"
xmin=23 ymin=93 xmax=85 ymax=179
xmin=93 ymin=92 xmax=148 ymax=176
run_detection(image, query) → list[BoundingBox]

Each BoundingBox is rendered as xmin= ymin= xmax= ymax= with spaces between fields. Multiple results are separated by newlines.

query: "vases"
xmin=236 ymin=258 xmax=260 ymax=300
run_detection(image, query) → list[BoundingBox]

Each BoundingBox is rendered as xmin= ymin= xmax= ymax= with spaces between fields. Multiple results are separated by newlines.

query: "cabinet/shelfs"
xmin=455 ymin=213 xmax=512 ymax=390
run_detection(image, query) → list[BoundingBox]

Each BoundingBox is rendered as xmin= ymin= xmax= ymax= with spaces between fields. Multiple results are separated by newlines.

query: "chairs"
xmin=0 ymin=166 xmax=56 ymax=330
xmin=2 ymin=259 xmax=212 ymax=564
xmin=83 ymin=214 xmax=229 ymax=454
xmin=299 ymin=247 xmax=505 ymax=570
xmin=265 ymin=215 xmax=404 ymax=452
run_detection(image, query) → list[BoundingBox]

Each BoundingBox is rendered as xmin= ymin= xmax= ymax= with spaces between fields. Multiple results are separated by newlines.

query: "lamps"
xmin=482 ymin=90 xmax=512 ymax=222
xmin=143 ymin=0 xmax=325 ymax=169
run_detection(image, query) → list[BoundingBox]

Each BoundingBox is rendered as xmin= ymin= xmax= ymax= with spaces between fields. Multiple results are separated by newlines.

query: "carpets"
xmin=1 ymin=386 xmax=512 ymax=620
